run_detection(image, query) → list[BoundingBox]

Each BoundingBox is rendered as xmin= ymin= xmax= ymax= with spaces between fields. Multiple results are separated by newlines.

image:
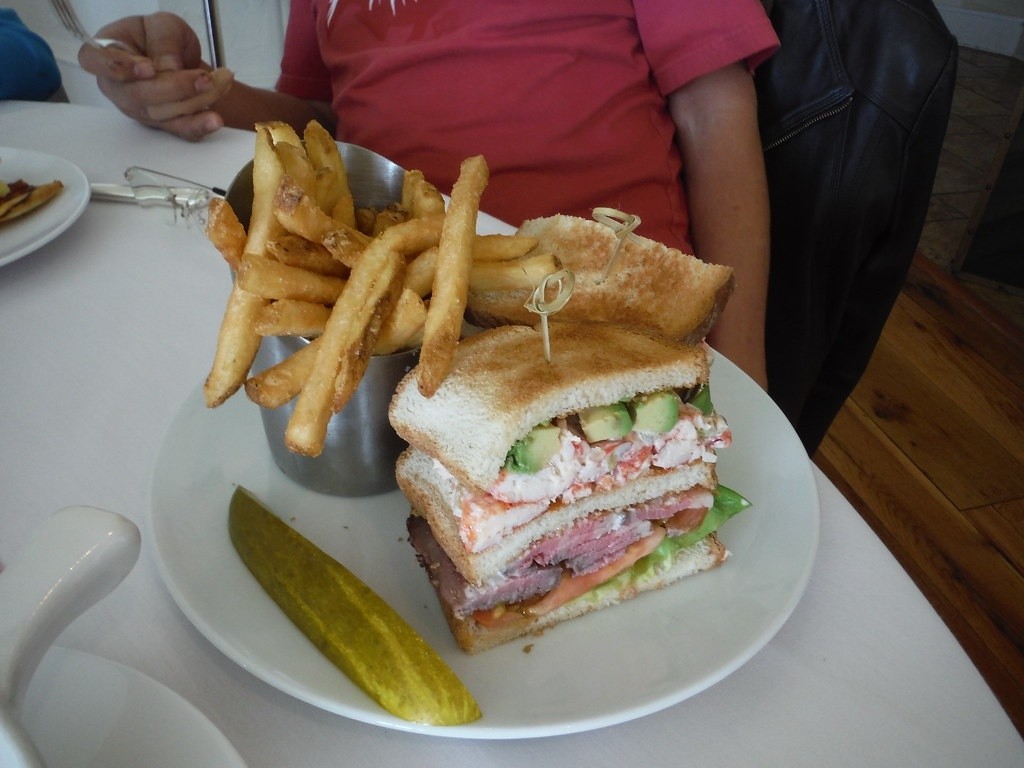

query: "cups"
xmin=222 ymin=141 xmax=421 ymax=497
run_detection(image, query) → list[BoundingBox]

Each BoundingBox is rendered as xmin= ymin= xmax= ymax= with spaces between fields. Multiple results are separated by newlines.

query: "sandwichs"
xmin=386 ymin=320 xmax=747 ymax=654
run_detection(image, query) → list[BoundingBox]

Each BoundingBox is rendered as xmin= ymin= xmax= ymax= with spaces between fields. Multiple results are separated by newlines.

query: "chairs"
xmin=737 ymin=0 xmax=965 ymax=457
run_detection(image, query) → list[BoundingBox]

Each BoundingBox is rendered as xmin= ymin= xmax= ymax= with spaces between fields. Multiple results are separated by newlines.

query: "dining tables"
xmin=0 ymin=98 xmax=1024 ymax=768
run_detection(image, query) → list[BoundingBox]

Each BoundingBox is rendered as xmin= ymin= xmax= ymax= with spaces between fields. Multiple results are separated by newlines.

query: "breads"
xmin=465 ymin=223 xmax=731 ymax=345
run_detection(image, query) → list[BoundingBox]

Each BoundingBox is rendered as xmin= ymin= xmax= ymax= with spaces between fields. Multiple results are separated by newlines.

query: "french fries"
xmin=206 ymin=120 xmax=564 ymax=460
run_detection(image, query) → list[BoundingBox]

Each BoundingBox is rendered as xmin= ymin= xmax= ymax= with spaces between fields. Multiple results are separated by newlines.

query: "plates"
xmin=0 ymin=648 xmax=248 ymax=768
xmin=147 ymin=342 xmax=821 ymax=739
xmin=0 ymin=145 xmax=91 ymax=268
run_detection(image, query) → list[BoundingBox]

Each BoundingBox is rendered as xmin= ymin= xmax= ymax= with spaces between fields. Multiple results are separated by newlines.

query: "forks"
xmin=50 ymin=0 xmax=212 ymax=112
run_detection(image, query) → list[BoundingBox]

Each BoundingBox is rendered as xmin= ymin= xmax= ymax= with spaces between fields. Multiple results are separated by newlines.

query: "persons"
xmin=76 ymin=0 xmax=782 ymax=397
